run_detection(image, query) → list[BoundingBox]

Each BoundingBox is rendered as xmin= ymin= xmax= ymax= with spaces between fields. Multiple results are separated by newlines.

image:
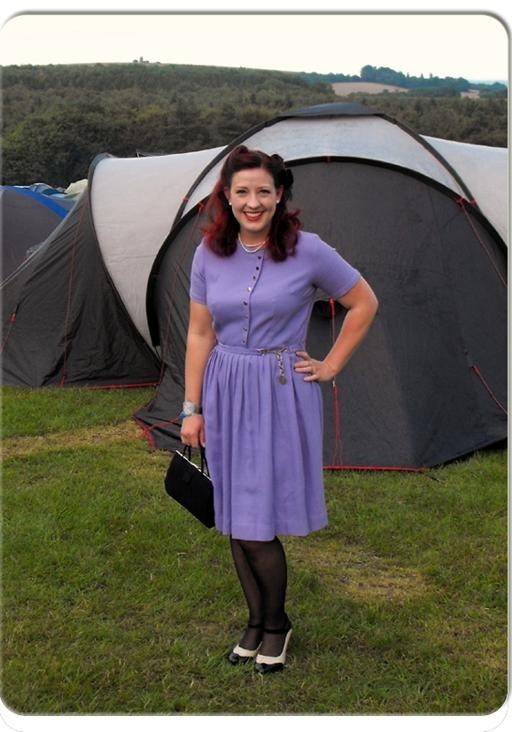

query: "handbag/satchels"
xmin=164 ymin=450 xmax=215 ymax=529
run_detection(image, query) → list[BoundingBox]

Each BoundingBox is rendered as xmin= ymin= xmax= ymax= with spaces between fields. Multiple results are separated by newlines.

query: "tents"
xmin=0 ymin=99 xmax=509 ymax=468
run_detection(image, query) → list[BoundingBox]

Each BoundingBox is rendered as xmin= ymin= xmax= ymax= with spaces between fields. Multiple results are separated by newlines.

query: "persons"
xmin=179 ymin=146 xmax=381 ymax=674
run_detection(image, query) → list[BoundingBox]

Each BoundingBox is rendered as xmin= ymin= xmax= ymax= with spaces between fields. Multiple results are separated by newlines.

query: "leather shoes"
xmin=229 ymin=624 xmax=262 ymax=665
xmin=255 ymin=614 xmax=293 ymax=675
